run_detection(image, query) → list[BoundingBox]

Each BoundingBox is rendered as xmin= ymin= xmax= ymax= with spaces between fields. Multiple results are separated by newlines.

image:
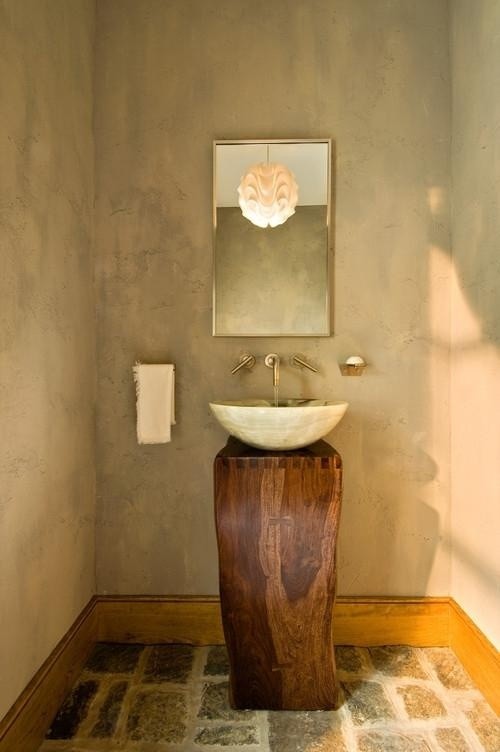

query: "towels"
xmin=132 ymin=360 xmax=177 ymax=445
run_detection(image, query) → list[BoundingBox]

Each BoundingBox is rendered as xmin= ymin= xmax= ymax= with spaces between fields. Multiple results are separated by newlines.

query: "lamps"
xmin=237 ymin=143 xmax=298 ymax=228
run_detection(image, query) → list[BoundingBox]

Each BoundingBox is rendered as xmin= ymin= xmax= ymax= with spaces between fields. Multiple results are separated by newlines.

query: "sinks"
xmin=206 ymin=395 xmax=351 ymax=451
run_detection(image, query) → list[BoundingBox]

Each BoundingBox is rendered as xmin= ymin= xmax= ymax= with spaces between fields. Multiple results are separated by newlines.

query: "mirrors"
xmin=213 ymin=139 xmax=333 ymax=337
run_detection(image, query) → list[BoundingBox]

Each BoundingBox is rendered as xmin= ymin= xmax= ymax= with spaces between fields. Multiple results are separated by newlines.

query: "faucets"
xmin=264 ymin=353 xmax=282 ymax=387
